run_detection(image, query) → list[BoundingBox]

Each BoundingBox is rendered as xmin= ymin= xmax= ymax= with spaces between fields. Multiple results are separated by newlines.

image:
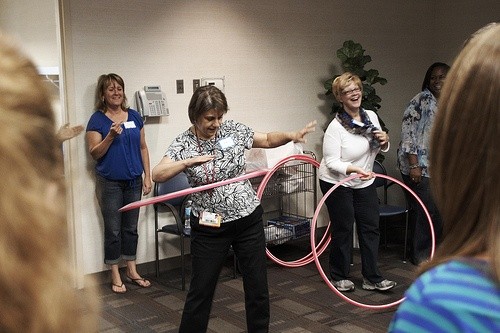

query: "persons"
xmin=152 ymin=86 xmax=316 ymax=333
xmin=86 ymin=74 xmax=151 ymax=294
xmin=397 ymin=61 xmax=451 ymax=265
xmin=318 ymin=72 xmax=397 ymax=292
xmin=0 ymin=38 xmax=101 ymax=332
xmin=388 ymin=23 xmax=500 ymax=333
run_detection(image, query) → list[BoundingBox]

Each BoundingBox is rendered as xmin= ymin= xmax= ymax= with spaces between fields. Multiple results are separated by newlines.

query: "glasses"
xmin=342 ymin=86 xmax=361 ymax=95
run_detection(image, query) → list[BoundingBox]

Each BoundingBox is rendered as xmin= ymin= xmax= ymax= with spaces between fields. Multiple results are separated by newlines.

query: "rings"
xmin=412 ymin=177 xmax=414 ymax=180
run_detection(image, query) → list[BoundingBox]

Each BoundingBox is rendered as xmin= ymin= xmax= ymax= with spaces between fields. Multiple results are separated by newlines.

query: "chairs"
xmin=154 ymin=173 xmax=237 ymax=290
xmin=373 ymin=160 xmax=409 ymax=264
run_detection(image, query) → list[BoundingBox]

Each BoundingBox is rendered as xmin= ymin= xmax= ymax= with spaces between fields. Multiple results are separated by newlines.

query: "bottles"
xmin=185 ymin=200 xmax=193 ymax=229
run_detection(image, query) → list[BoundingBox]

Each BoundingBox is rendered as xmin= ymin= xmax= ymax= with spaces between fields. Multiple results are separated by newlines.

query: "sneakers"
xmin=331 ymin=279 xmax=354 ymax=291
xmin=362 ymin=276 xmax=397 ymax=290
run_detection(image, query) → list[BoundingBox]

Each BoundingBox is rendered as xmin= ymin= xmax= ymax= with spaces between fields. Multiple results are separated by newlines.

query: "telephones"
xmin=134 ymin=86 xmax=170 ymax=118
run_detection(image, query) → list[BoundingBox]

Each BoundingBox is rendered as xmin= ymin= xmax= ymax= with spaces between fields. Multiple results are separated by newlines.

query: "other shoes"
xmin=412 ymin=256 xmax=426 ymax=265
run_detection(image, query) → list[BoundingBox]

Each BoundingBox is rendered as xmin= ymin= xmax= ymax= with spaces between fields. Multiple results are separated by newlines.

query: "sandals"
xmin=126 ymin=275 xmax=152 ymax=288
xmin=111 ymin=281 xmax=127 ymax=294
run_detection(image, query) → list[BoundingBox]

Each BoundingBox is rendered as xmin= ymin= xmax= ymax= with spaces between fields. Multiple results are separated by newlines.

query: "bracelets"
xmin=410 ymin=162 xmax=418 ymax=168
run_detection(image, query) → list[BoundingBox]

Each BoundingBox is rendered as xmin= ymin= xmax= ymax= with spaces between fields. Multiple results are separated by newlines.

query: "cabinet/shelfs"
xmin=248 ymin=151 xmax=317 ymax=251
